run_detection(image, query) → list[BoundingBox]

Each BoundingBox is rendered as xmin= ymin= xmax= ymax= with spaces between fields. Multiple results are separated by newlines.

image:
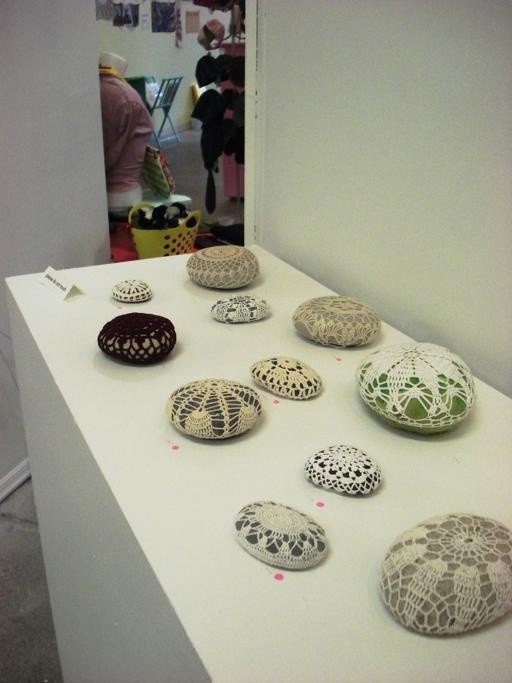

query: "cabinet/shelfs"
xmin=144 ymin=74 xmax=184 ymax=148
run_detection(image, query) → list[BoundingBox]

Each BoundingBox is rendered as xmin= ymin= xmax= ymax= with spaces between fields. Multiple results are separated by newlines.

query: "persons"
xmin=98 ymin=52 xmax=155 ymax=216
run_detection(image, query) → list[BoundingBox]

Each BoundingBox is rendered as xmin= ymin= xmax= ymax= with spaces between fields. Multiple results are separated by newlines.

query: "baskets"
xmin=128 ymin=204 xmax=201 ymax=258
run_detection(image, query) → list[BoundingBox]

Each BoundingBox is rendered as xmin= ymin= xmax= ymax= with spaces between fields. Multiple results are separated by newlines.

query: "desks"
xmin=5 ymin=244 xmax=512 ymax=682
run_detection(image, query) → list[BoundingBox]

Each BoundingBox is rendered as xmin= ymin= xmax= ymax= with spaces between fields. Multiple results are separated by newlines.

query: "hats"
xmin=197 ymin=20 xmax=224 ymax=50
xmin=191 ymin=54 xmax=244 ymax=170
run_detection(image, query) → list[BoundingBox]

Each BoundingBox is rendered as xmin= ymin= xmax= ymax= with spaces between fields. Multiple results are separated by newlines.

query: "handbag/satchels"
xmin=145 ymin=145 xmax=176 ymax=199
